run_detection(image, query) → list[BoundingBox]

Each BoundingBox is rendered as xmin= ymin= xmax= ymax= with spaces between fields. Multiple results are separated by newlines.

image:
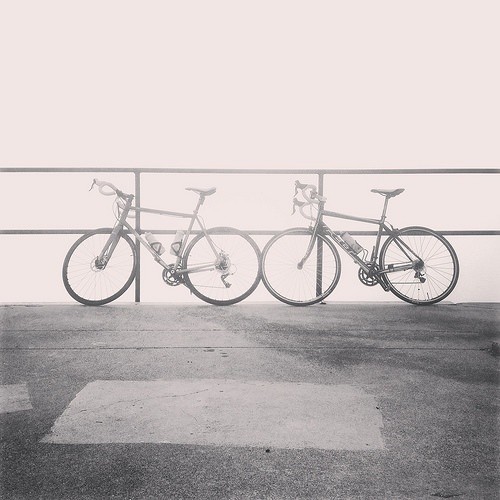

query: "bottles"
xmin=340 ymin=230 xmax=362 ymax=254
xmin=169 ymin=229 xmax=185 ymax=256
xmin=144 ymin=231 xmax=166 ymax=256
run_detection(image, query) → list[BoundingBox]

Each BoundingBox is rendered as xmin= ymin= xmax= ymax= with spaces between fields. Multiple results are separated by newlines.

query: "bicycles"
xmin=62 ymin=176 xmax=260 ymax=308
xmin=260 ymin=178 xmax=459 ymax=307
